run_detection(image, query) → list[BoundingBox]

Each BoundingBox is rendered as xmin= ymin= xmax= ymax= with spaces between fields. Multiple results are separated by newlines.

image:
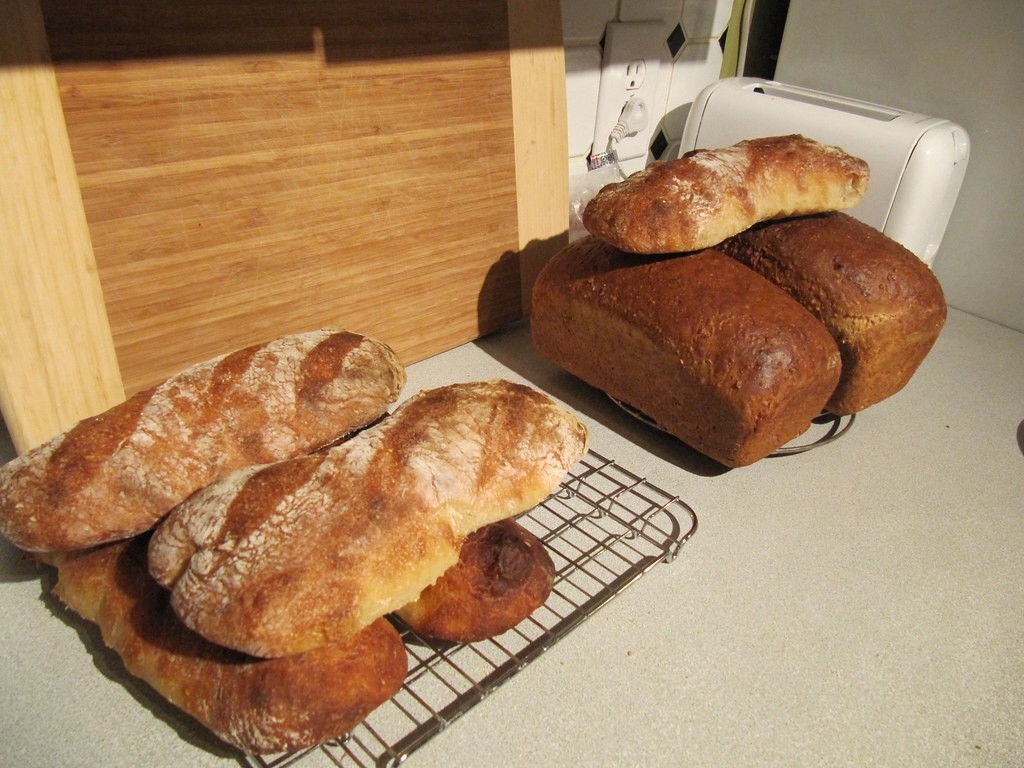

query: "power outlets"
xmin=590 ymin=18 xmax=667 ymax=168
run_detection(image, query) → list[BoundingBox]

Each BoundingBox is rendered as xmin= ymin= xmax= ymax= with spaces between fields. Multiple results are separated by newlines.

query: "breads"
xmin=147 ymin=375 xmax=588 ymax=659
xmin=0 ymin=326 xmax=406 ymax=555
xmin=681 ymin=148 xmax=949 ymax=417
xmin=41 ymin=527 xmax=408 ymax=752
xmin=582 ymin=132 xmax=871 ymax=254
xmin=531 ymin=231 xmax=843 ymax=468
xmin=390 ymin=514 xmax=555 ymax=643
